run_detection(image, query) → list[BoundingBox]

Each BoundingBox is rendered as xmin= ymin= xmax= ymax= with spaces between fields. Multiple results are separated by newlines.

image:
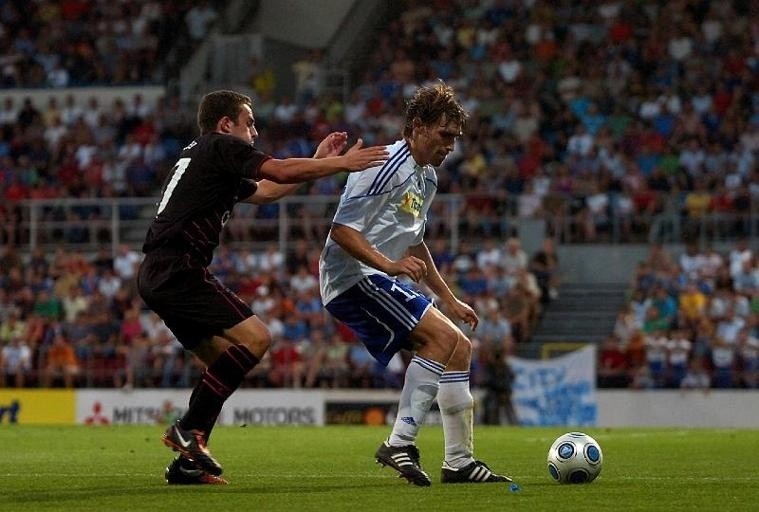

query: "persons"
xmin=479 ymin=340 xmax=518 ymax=427
xmin=314 ymin=78 xmax=515 ymax=487
xmin=135 ymin=89 xmax=390 ymax=488
xmin=1 ymin=1 xmax=758 ymax=397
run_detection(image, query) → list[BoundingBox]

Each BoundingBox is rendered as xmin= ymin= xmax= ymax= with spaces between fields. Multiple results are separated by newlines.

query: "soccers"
xmin=547 ymin=431 xmax=602 ymax=485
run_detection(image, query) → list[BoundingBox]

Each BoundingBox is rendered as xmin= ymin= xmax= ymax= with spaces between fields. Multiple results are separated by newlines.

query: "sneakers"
xmin=440 ymin=460 xmax=513 ymax=483
xmin=162 ymin=418 xmax=222 ymax=476
xmin=165 ymin=457 xmax=229 ymax=485
xmin=374 ymin=435 xmax=432 ymax=486
